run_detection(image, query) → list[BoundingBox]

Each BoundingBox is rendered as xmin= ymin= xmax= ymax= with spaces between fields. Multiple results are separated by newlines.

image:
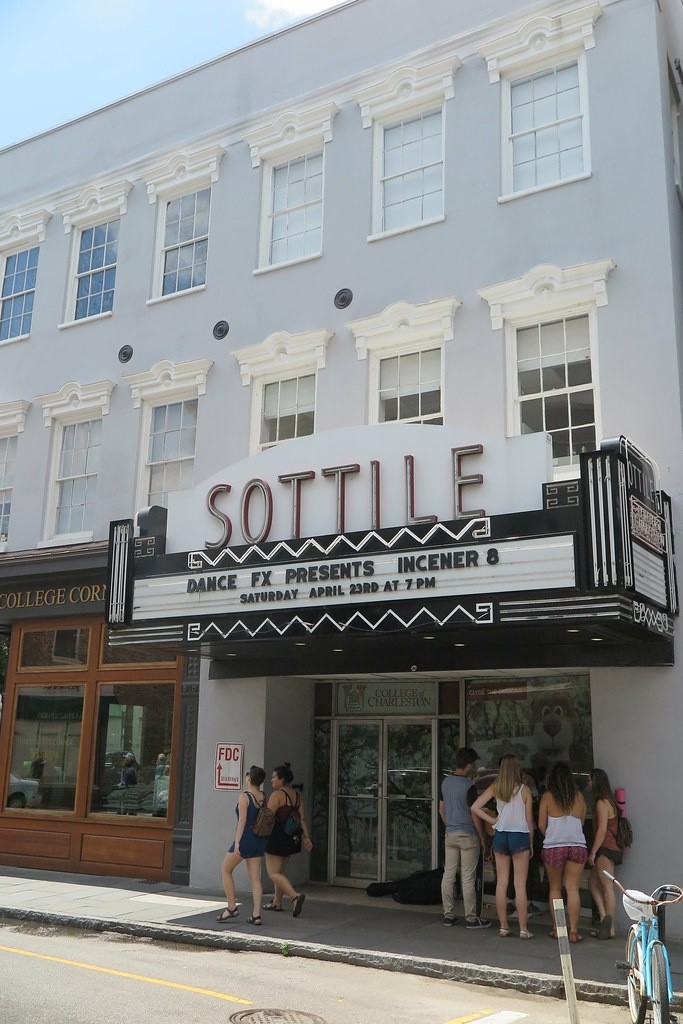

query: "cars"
xmin=372 ymin=765 xmax=597 ymax=815
xmin=90 ymin=750 xmax=171 ymax=818
xmin=4 ymin=773 xmax=44 ymax=810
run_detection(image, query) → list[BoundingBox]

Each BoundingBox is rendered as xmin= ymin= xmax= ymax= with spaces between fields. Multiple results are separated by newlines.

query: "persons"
xmin=262 ymin=761 xmax=313 ymax=918
xmin=120 ymin=753 xmax=142 ymax=786
xmin=216 ymin=765 xmax=267 ymax=925
xmin=585 ymin=768 xmax=623 ymax=940
xmin=471 ymin=755 xmax=535 ymax=940
xmin=30 ymin=752 xmax=44 ymax=781
xmin=438 ymin=748 xmax=492 ymax=928
xmin=155 ymin=754 xmax=169 ymax=776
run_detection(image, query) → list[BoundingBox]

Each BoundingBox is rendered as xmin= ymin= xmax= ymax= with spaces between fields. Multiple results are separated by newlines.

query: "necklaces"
xmin=538 ymin=761 xmax=587 ymax=943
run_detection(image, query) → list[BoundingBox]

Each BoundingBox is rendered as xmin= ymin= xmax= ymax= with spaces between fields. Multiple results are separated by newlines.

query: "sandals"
xmin=263 ymin=901 xmax=283 ymax=911
xmin=292 ymin=894 xmax=305 ymax=918
xmin=246 ymin=915 xmax=262 ymax=925
xmin=216 ymin=907 xmax=239 ymax=921
xmin=569 ymin=931 xmax=584 ymax=943
xmin=550 ymin=929 xmax=558 ymax=940
xmin=500 ymin=927 xmax=512 ymax=937
xmin=520 ymin=930 xmax=534 ymax=940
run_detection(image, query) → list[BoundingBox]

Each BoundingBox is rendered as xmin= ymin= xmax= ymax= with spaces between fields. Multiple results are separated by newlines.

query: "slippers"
xmin=609 ymin=935 xmax=616 ymax=939
xmin=598 ymin=915 xmax=612 ymax=939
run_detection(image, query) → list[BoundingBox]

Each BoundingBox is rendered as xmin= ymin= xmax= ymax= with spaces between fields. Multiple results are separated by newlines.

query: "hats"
xmin=123 ymin=752 xmax=135 ymax=759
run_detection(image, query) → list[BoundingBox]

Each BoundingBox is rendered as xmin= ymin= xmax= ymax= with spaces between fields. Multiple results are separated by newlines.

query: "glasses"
xmin=246 ymin=772 xmax=250 ymax=779
xmin=586 ymin=779 xmax=593 ymax=783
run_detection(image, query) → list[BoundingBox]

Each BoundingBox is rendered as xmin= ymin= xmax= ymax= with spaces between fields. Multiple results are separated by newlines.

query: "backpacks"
xmin=246 ymin=790 xmax=273 ymax=836
xmin=608 ymin=796 xmax=633 ymax=849
xmin=274 ymin=789 xmax=302 ymax=854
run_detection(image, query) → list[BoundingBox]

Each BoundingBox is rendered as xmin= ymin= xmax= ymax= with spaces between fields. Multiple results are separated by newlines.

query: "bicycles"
xmin=602 ymin=868 xmax=683 ymax=1024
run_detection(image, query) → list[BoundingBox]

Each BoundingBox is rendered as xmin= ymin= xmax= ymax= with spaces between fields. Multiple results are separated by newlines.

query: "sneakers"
xmin=443 ymin=917 xmax=459 ymax=926
xmin=465 ymin=916 xmax=491 ymax=929
